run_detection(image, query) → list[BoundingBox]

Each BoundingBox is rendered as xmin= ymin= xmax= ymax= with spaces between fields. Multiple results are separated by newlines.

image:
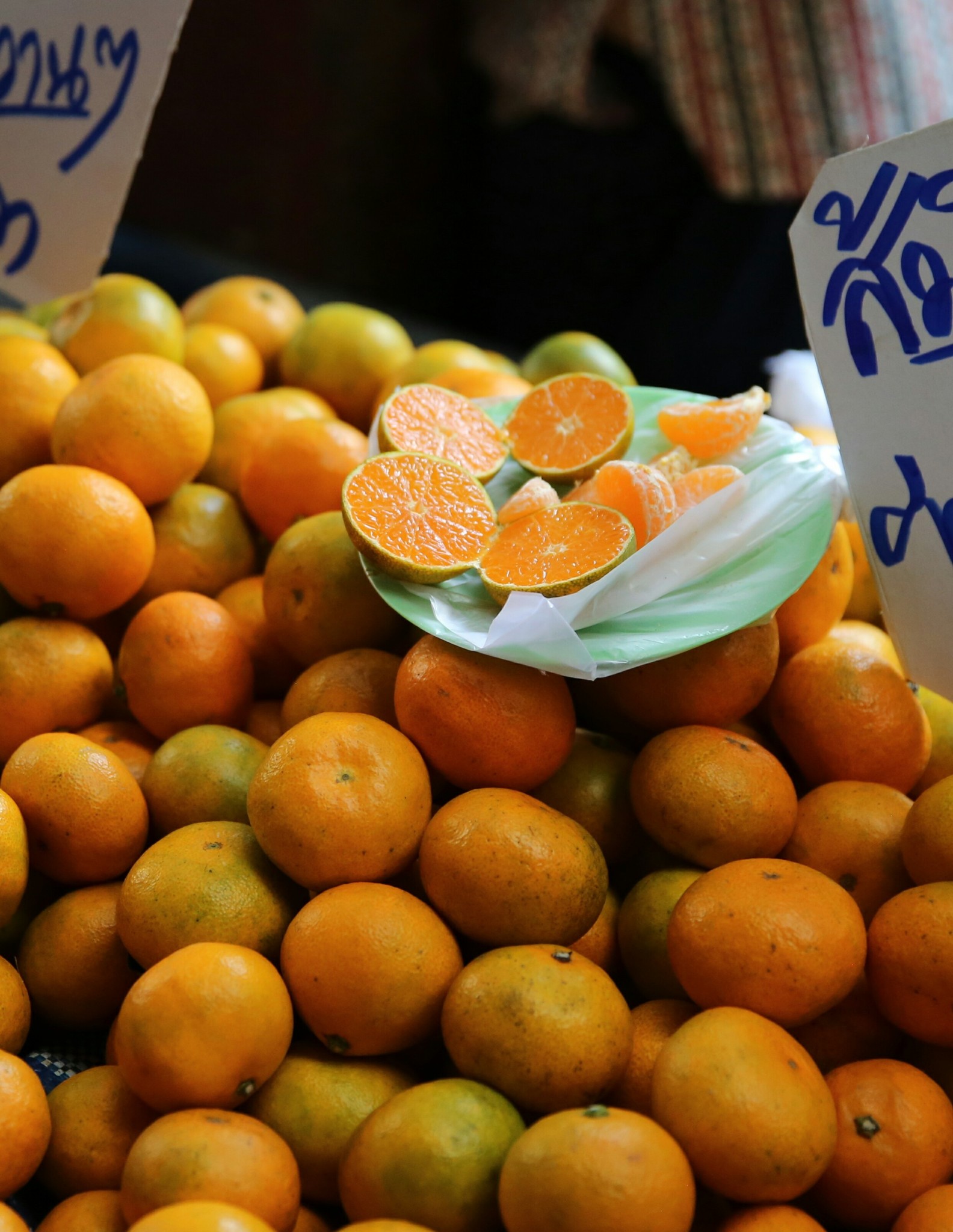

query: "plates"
xmin=356 ymin=385 xmax=832 ymax=666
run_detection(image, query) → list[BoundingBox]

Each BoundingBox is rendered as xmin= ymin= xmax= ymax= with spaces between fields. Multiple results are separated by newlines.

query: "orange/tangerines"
xmin=0 ymin=275 xmax=953 ymax=1232
